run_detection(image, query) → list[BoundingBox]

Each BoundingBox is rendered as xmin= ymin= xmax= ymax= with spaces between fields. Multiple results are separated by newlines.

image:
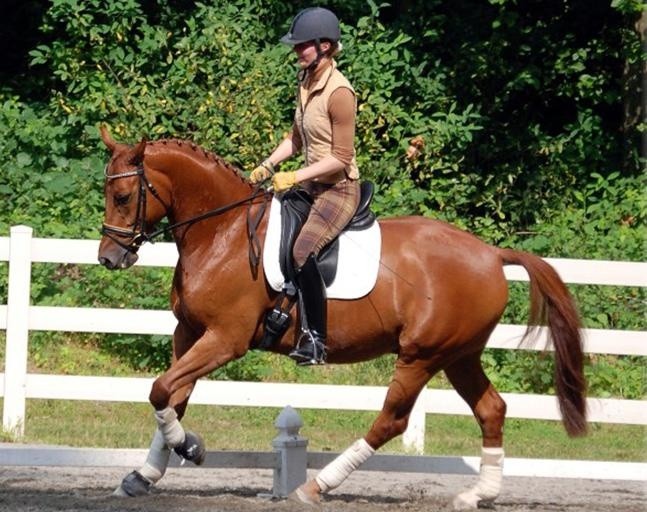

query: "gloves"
xmin=248 ymin=159 xmax=296 ymax=192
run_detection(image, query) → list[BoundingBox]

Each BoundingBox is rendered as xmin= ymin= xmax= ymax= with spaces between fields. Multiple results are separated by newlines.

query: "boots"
xmin=290 ymin=254 xmax=327 ymax=362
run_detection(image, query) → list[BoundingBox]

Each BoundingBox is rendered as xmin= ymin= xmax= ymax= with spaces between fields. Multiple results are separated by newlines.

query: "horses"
xmin=91 ymin=122 xmax=590 ymax=512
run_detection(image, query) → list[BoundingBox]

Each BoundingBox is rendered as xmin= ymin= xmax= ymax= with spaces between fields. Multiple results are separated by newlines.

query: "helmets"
xmin=281 ymin=7 xmax=339 ymax=44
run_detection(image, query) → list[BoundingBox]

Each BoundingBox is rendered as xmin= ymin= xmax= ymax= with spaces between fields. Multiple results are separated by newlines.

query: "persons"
xmin=249 ymin=6 xmax=363 ymax=365
